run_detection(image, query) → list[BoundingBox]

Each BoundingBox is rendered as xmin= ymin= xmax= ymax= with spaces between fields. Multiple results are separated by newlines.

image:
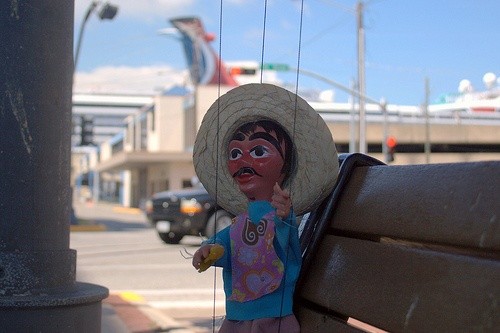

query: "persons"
xmin=191 ymin=83 xmax=339 ymax=333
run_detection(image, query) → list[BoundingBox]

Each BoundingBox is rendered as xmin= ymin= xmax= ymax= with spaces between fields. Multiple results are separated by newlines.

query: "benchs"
xmin=293 ymin=152 xmax=500 ymax=333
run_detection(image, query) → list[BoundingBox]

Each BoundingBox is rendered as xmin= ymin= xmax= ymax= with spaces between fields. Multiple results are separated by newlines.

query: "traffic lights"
xmin=231 ymin=66 xmax=256 ymax=76
xmin=386 ymin=136 xmax=397 ymax=148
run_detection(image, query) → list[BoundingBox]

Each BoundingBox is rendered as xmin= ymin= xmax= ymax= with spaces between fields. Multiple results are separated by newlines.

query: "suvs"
xmin=146 ymin=181 xmax=236 ymax=244
